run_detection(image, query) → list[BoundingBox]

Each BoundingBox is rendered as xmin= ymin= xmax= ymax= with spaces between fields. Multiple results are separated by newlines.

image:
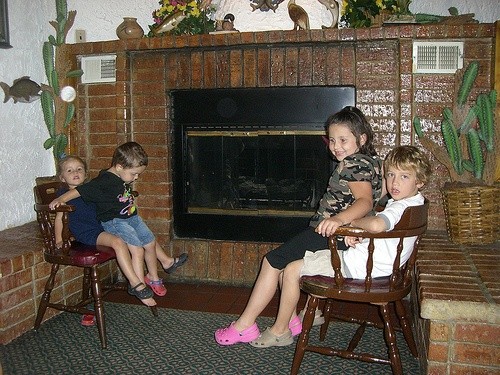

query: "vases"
xmin=116 ymin=18 xmax=144 ymax=39
xmin=363 ymin=9 xmax=392 ymax=28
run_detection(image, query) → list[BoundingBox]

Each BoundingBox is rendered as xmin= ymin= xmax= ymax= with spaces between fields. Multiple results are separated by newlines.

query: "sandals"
xmin=214 ymin=321 xmax=260 ymax=345
xmin=298 ymin=310 xmax=325 ymax=326
xmin=127 ymin=282 xmax=154 ymax=299
xmin=164 ymin=252 xmax=188 ymax=274
xmin=288 ymin=316 xmax=302 ymax=336
xmin=249 ymin=327 xmax=294 ymax=348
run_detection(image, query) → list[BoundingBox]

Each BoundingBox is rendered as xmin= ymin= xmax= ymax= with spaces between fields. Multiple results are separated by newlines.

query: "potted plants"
xmin=413 ymin=61 xmax=500 ymax=246
xmin=34 ymin=0 xmax=84 ymax=227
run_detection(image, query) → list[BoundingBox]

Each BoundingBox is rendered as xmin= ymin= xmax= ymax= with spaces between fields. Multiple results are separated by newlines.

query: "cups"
xmin=75 ymin=30 xmax=86 ymax=43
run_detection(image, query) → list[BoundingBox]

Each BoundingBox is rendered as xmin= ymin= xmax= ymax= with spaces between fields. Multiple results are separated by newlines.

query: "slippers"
xmin=144 ymin=275 xmax=168 ymax=296
xmin=81 ymin=312 xmax=95 ymax=326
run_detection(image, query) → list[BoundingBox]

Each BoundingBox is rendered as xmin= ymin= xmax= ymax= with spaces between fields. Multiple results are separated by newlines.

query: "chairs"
xmin=292 ymin=198 xmax=429 ymax=375
xmin=34 ymin=182 xmax=157 ymax=350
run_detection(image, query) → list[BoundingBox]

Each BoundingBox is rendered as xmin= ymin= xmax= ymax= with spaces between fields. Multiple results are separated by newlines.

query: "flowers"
xmin=144 ymin=0 xmax=216 ymax=37
xmin=341 ymin=0 xmax=411 ymax=29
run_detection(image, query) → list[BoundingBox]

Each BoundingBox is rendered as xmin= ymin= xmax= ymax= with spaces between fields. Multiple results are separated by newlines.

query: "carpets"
xmin=0 ymin=301 xmax=422 ymax=375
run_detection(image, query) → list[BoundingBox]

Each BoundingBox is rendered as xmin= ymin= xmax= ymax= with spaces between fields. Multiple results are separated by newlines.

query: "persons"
xmin=49 ymin=142 xmax=188 ymax=299
xmin=214 ymin=106 xmax=431 ymax=348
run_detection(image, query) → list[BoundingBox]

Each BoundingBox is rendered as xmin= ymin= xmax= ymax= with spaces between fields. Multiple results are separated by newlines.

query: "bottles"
xmin=116 ymin=17 xmax=144 ymax=40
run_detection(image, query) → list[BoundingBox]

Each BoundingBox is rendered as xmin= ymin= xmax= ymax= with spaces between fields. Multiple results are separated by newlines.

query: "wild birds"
xmin=288 ymin=1 xmax=310 ymax=30
